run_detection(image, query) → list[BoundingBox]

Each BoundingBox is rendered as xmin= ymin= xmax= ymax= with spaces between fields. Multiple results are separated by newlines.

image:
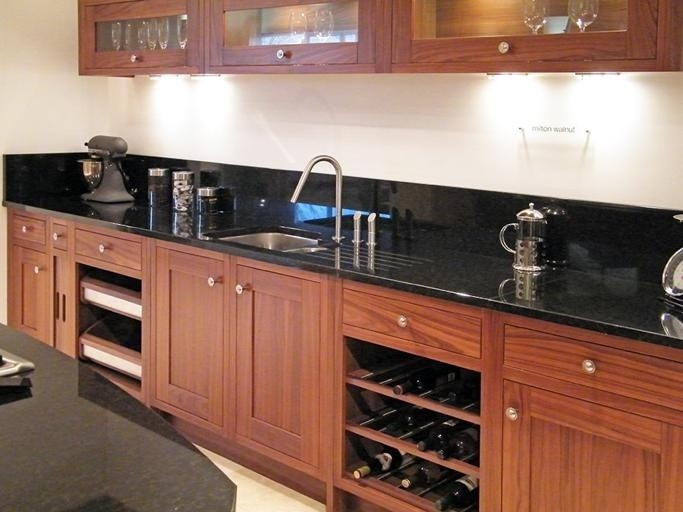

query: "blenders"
xmin=80 ymin=201 xmax=134 ymax=227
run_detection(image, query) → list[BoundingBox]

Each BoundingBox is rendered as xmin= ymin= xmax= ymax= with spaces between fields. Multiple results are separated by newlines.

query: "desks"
xmin=1 ymin=321 xmax=236 ymax=510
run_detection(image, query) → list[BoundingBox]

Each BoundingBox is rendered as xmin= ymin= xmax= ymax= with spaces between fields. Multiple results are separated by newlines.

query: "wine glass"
xmin=567 ymin=0 xmax=600 ymax=31
xmin=524 ymin=0 xmax=547 ymax=33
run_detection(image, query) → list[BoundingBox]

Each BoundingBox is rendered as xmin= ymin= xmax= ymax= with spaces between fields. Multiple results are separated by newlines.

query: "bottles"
xmin=146 ymin=168 xmax=169 ymax=206
xmin=350 ymin=366 xmax=480 ymax=512
xmin=172 ymin=171 xmax=195 ymax=214
xmin=199 ymin=169 xmax=220 ymax=187
xmin=194 ymin=186 xmax=221 ymax=215
xmin=217 ymin=185 xmax=237 ymax=225
xmin=145 ymin=206 xmax=216 ymax=243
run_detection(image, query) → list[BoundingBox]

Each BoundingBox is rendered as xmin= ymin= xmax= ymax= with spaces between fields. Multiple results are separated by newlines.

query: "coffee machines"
xmin=76 ymin=135 xmax=135 ymax=202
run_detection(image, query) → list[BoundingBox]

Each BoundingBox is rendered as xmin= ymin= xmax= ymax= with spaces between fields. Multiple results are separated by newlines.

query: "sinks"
xmin=200 ymin=223 xmax=331 ymax=255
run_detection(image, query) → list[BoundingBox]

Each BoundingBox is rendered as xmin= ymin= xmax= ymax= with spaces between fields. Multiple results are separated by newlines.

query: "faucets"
xmin=289 ymin=154 xmax=346 ymax=243
xmin=334 ymin=247 xmax=341 ymax=271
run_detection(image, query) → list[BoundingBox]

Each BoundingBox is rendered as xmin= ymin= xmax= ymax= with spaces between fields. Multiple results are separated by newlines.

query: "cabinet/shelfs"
xmin=384 ymin=0 xmax=683 ymax=74
xmin=152 ymin=240 xmax=325 ymax=508
xmin=205 ymin=1 xmax=381 ymax=75
xmin=325 ymin=272 xmax=492 ymax=512
xmin=490 ymin=305 xmax=681 ymax=512
xmin=77 ymin=0 xmax=199 ymax=76
xmin=72 ymin=222 xmax=149 ymax=409
xmin=4 ymin=201 xmax=75 ymax=356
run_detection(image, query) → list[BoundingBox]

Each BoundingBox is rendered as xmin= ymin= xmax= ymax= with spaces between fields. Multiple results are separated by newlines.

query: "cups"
xmin=108 ymin=13 xmax=187 ymax=50
xmin=496 ymin=268 xmax=545 ymax=310
xmin=497 ymin=201 xmax=549 ymax=274
xmin=540 ymin=203 xmax=571 ymax=267
xmin=310 ymin=7 xmax=332 ymax=41
xmin=287 ymin=10 xmax=310 ymax=45
